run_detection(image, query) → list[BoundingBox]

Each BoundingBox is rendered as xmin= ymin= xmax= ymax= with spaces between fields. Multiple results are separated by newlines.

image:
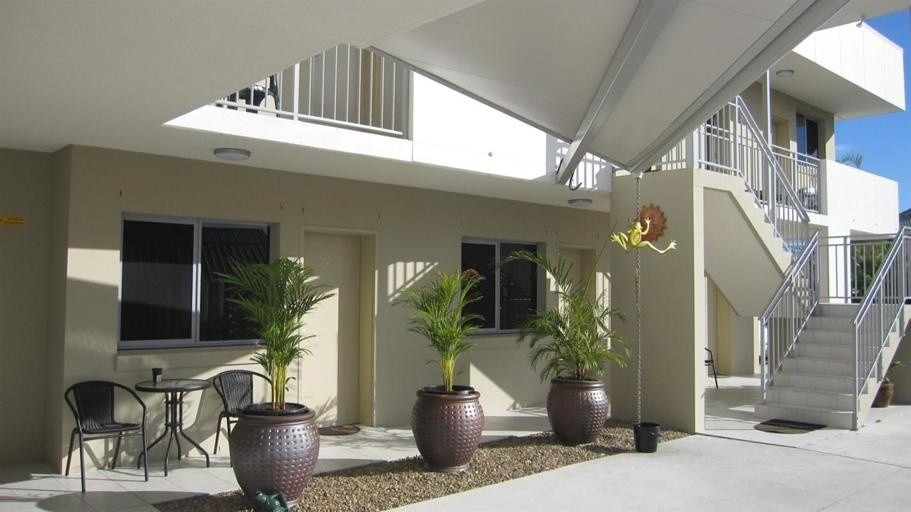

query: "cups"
xmin=152 ymin=367 xmax=162 ymax=383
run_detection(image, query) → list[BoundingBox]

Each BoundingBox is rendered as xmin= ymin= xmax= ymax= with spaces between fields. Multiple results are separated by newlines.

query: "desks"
xmin=135 ymin=379 xmax=211 ymax=476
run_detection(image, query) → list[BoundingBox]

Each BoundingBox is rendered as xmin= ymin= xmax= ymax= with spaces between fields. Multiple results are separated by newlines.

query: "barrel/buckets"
xmin=632 ymin=422 xmax=663 ymax=452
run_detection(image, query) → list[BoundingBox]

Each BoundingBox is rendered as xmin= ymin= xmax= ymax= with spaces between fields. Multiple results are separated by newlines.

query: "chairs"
xmin=64 ymin=380 xmax=148 ymax=494
xmin=207 ymin=370 xmax=275 ymax=469
xmin=704 ymin=346 xmax=718 ymax=390
xmin=215 ymin=72 xmax=280 ymax=117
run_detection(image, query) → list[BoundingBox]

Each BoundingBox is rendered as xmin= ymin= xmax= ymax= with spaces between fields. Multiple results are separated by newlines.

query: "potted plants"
xmin=388 ymin=265 xmax=489 ymax=473
xmin=489 ymin=216 xmax=632 ymax=446
xmin=871 ymin=359 xmax=906 ymax=407
xmin=212 ymin=251 xmax=337 ymax=512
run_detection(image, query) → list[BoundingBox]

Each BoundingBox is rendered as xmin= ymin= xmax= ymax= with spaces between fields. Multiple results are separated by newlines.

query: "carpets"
xmin=752 ymin=419 xmax=826 ymax=434
